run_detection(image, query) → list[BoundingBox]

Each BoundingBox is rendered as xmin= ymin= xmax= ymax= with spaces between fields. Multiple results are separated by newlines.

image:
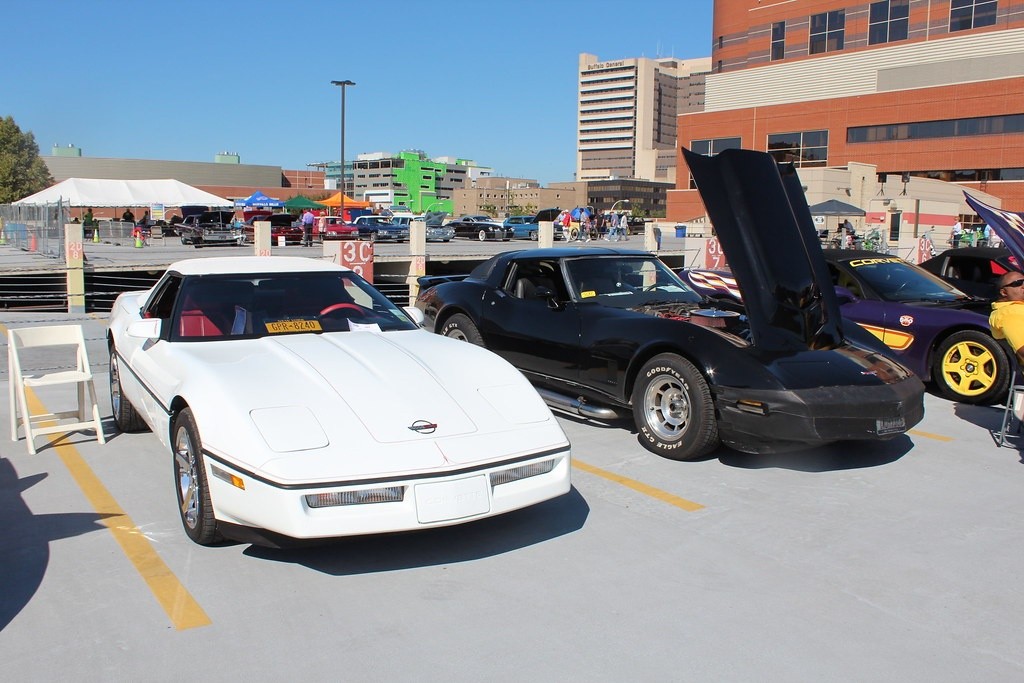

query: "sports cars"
xmin=415 ymin=147 xmax=927 ymax=463
xmin=909 ymin=191 xmax=1024 ymax=300
xmin=675 ymin=249 xmax=1024 ymax=407
xmin=106 ymin=255 xmax=572 ymax=548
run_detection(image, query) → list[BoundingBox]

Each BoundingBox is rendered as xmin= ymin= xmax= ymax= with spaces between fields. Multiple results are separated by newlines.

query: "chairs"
xmin=997 ymin=337 xmax=1024 ymax=446
xmin=517 ymin=266 xmax=559 ymax=299
xmin=579 ymin=261 xmax=616 ymax=295
xmin=179 ymin=291 xmax=225 ymax=336
xmin=8 ymin=325 xmax=107 ymax=455
xmin=150 ymin=226 xmax=166 ymax=247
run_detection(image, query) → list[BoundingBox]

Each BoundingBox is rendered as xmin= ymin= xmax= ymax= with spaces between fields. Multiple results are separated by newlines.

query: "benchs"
xmin=687 ymin=233 xmax=705 ymax=238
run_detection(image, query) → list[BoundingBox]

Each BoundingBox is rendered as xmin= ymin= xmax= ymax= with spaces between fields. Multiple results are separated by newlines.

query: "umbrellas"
xmin=809 ymin=199 xmax=866 ymax=229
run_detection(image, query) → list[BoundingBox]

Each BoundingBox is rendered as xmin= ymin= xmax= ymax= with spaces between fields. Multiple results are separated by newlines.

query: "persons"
xmin=319 ymin=212 xmax=326 ymax=241
xmin=379 ymin=205 xmax=383 ymax=210
xmin=85 ymin=209 xmax=93 ymax=225
xmin=949 ymin=217 xmax=961 ymax=248
xmin=983 ymin=224 xmax=994 ymax=240
xmin=605 ymin=212 xmax=618 ymax=242
xmin=73 ymin=218 xmax=80 ymax=223
xmin=93 ymin=219 xmax=99 ymax=226
xmin=844 ymin=220 xmax=853 ymax=229
xmin=123 ymin=209 xmax=134 ymax=222
xmin=594 ymin=209 xmax=604 ymax=241
xmin=558 ymin=210 xmax=571 ymax=242
xmin=618 ymin=212 xmax=629 ymax=241
xmin=302 ymin=209 xmax=314 ymax=247
xmin=575 ymin=208 xmax=590 ymax=243
xmin=144 ymin=211 xmax=148 ymax=219
xmin=989 ymin=271 xmax=1024 ymax=365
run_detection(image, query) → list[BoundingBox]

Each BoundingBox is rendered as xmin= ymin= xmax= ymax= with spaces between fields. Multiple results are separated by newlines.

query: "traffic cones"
xmin=134 ymin=231 xmax=144 ymax=248
xmin=0 ymin=231 xmax=8 ymax=246
xmin=29 ymin=234 xmax=37 ymax=252
xmin=93 ymin=228 xmax=100 ymax=243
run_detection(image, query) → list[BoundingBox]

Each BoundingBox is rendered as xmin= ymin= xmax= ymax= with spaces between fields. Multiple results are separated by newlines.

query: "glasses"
xmin=1001 ymin=279 xmax=1024 ymax=287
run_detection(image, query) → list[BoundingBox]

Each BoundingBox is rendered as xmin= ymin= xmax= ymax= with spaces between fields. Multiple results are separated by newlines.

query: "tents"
xmin=12 ymin=179 xmax=235 ymax=218
xmin=235 ymin=190 xmax=376 ymax=216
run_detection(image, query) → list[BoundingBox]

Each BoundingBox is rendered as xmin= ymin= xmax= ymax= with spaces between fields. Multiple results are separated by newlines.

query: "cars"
xmin=348 ymin=203 xmax=458 ymax=243
xmin=502 ymin=208 xmax=566 ymax=241
xmin=297 ymin=216 xmax=359 ymax=240
xmin=346 ymin=215 xmax=411 ymax=244
xmin=146 ymin=208 xmax=305 ymax=249
xmin=553 ymin=204 xmax=653 ymax=241
xmin=444 ymin=214 xmax=516 ymax=243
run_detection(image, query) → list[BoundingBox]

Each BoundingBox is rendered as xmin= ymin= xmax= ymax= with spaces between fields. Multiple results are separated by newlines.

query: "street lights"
xmin=610 ymin=199 xmax=630 ymax=211
xmin=330 ymin=79 xmax=357 ymax=219
